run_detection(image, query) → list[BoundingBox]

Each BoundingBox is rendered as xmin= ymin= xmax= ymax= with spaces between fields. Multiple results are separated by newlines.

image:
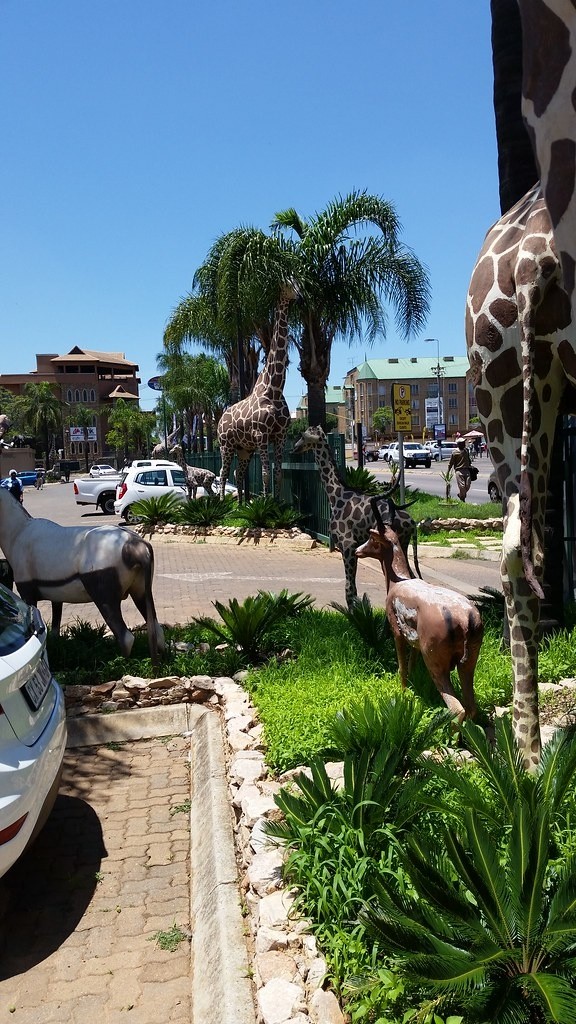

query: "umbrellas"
xmin=463 ymin=430 xmax=484 ymax=437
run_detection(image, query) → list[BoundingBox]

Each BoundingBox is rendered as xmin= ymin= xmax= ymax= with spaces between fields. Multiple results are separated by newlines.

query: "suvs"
xmin=377 ymin=444 xmax=389 ymax=462
xmin=387 ymin=442 xmax=432 ymax=469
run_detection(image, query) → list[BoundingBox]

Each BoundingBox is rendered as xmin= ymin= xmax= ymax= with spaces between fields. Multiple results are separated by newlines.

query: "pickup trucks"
xmin=72 ymin=458 xmax=188 ymax=516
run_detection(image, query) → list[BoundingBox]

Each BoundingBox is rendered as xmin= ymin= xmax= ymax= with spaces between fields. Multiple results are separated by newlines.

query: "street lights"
xmin=422 ymin=338 xmax=443 ymax=463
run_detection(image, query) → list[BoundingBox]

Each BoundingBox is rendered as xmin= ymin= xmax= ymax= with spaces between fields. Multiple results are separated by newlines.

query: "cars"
xmin=0 ymin=583 xmax=69 ymax=882
xmin=486 ymin=469 xmax=503 ymax=503
xmin=424 ymin=439 xmax=461 ymax=462
xmin=113 ymin=465 xmax=237 ymax=526
xmin=88 ymin=464 xmax=117 ymax=478
xmin=0 ymin=470 xmax=45 ymax=488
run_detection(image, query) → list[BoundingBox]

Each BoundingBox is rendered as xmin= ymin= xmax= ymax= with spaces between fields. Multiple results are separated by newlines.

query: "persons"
xmin=472 ymin=438 xmax=480 ymax=457
xmin=446 ymin=437 xmax=472 ymax=503
xmin=64 ymin=465 xmax=70 ymax=482
xmin=36 ymin=469 xmax=43 ymax=490
xmin=0 ymin=469 xmax=24 ymax=505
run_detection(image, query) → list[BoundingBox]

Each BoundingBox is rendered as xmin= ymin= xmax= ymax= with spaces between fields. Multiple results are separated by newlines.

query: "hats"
xmin=457 ymin=438 xmax=467 ymax=444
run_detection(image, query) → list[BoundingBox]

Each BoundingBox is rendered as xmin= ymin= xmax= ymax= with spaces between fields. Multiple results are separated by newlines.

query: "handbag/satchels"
xmin=469 ymin=466 xmax=478 ymax=481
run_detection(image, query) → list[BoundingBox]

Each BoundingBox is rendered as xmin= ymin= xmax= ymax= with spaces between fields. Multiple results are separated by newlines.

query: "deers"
xmin=356 ymin=470 xmax=484 ymax=749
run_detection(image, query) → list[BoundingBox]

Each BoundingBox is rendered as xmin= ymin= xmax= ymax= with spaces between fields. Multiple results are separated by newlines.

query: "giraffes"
xmin=218 ymin=284 xmax=300 ymax=502
xmin=467 ymin=1 xmax=574 ymax=725
xmin=168 ymin=444 xmax=215 ymax=499
xmin=290 ymin=423 xmax=423 ymax=610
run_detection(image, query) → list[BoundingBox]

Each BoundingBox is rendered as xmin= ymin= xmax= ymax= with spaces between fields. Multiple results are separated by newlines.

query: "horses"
xmin=0 ymin=486 xmax=169 ymax=666
